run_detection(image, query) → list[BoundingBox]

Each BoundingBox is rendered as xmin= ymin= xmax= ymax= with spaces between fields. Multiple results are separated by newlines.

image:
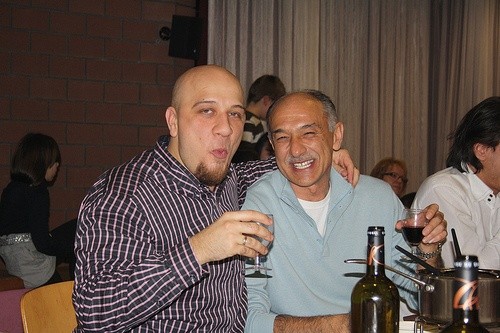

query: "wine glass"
xmin=402 ymin=209 xmax=429 ymax=261
xmin=242 ymin=214 xmax=274 ymax=269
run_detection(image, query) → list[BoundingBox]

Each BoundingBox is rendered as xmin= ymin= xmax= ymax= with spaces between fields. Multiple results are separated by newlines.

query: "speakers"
xmin=168 ymin=14 xmax=204 ymax=59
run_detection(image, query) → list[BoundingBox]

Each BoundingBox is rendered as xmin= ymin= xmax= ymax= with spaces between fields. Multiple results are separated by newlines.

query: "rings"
xmin=243 ymin=236 xmax=247 ymax=246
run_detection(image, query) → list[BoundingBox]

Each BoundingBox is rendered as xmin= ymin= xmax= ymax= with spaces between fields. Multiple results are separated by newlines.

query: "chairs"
xmin=0 ymin=281 xmax=76 ymax=333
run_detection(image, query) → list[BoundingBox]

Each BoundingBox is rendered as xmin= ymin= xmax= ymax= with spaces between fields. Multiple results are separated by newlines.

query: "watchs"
xmin=416 ymin=242 xmax=442 ymax=259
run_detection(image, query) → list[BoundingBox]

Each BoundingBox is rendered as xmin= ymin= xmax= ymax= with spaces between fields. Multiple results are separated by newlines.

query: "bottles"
xmin=350 ymin=226 xmax=400 ymax=333
xmin=439 ymin=255 xmax=492 ymax=333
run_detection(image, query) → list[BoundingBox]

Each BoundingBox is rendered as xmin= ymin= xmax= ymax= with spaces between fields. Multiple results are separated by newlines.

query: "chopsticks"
xmin=395 ymin=228 xmax=462 ymax=276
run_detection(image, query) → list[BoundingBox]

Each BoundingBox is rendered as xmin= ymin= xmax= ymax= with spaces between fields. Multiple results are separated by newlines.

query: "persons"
xmin=409 ymin=96 xmax=500 ymax=270
xmin=72 ymin=65 xmax=359 ymax=333
xmin=370 ymin=156 xmax=417 ymax=209
xmin=231 ymin=74 xmax=286 ymax=163
xmin=239 ymin=89 xmax=448 ymax=333
xmin=0 ymin=132 xmax=77 ymax=289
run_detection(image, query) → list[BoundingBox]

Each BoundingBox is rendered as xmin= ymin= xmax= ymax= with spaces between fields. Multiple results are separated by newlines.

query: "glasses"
xmin=383 ymin=171 xmax=408 ymax=184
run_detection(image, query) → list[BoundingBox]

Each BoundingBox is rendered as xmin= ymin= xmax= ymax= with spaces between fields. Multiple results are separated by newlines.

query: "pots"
xmin=344 ymin=259 xmax=500 ymax=325
